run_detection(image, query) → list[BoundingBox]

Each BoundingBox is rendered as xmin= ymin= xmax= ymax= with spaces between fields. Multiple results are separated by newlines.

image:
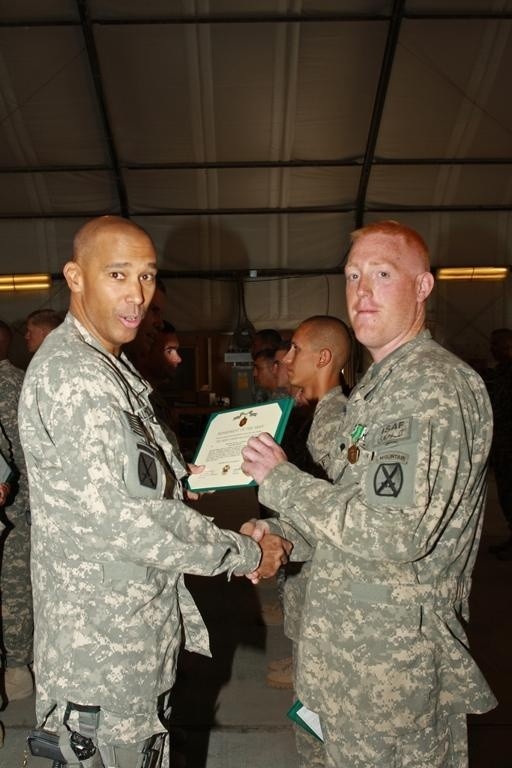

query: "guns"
xmin=28 ymin=729 xmax=95 ymax=763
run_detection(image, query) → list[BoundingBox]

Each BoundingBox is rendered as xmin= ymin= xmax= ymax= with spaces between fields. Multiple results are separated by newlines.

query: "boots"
xmin=4 ymin=665 xmax=33 ymax=702
xmin=263 ymin=602 xmax=283 ymax=625
xmin=264 ymin=656 xmax=293 ymax=688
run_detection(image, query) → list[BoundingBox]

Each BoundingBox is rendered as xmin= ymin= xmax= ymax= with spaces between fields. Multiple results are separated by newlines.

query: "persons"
xmin=485 ymin=328 xmax=510 ymax=560
xmin=239 ymin=220 xmax=499 ymax=768
xmin=17 ymin=215 xmax=294 ymax=767
xmin=2 ymin=275 xmax=182 ymax=767
xmin=251 ymin=315 xmax=355 ymax=689
